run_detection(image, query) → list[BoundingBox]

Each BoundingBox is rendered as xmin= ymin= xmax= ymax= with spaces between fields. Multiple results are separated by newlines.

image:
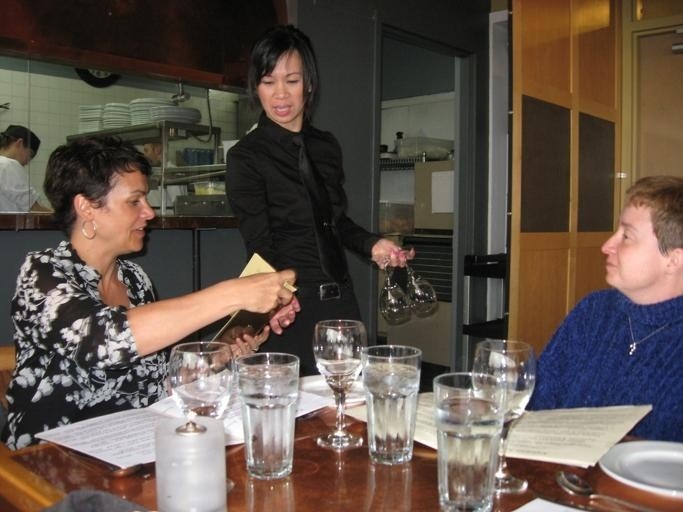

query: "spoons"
xmin=553 ymin=469 xmax=661 ymax=511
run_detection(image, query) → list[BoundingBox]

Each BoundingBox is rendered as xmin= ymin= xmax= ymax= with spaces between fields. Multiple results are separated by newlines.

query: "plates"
xmin=78 ymin=98 xmax=201 ymax=133
xmin=595 ymin=439 xmax=683 ymax=497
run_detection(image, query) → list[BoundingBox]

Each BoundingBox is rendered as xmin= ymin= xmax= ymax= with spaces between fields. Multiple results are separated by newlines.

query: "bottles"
xmin=185 ymin=147 xmax=214 ymax=166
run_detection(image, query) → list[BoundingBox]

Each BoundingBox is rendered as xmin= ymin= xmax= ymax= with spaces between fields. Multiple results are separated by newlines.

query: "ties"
xmin=290 ymin=134 xmax=349 ymax=283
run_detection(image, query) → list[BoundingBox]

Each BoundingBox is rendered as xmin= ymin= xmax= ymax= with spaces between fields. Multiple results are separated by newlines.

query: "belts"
xmin=289 ymin=277 xmax=351 ymax=303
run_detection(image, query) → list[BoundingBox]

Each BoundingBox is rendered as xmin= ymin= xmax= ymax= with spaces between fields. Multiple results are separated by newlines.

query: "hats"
xmin=5 ymin=125 xmax=41 ymax=151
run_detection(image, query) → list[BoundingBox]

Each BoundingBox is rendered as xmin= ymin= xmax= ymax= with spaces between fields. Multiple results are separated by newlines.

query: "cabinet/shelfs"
xmin=66 ymin=119 xmax=222 ymax=217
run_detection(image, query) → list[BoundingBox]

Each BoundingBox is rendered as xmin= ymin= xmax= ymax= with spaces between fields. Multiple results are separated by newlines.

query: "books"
xmin=32 ymin=364 xmax=334 ymax=471
xmin=205 ymin=252 xmax=297 ymax=350
xmin=340 ymin=379 xmax=655 ymax=469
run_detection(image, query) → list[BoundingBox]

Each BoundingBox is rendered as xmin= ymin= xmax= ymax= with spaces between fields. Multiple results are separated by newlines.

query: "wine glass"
xmin=166 ymin=338 xmax=235 ymax=497
xmin=472 ymin=338 xmax=537 ymax=495
xmin=373 ymin=246 xmax=439 ymax=327
xmin=310 ymin=318 xmax=367 ymax=453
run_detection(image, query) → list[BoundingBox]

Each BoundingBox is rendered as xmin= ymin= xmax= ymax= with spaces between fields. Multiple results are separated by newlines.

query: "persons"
xmin=222 ymin=25 xmax=416 ymax=377
xmin=523 ymin=174 xmax=682 ymax=445
xmin=4 ymin=130 xmax=297 ymax=450
xmin=0 ymin=124 xmax=54 ymax=212
xmin=142 ymin=142 xmax=187 ymax=215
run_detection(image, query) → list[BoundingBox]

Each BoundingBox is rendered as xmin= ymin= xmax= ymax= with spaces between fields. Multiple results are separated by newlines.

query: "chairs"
xmin=0 ymin=344 xmax=17 ymax=414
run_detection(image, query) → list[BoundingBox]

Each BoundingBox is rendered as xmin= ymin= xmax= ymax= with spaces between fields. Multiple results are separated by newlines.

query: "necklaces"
xmin=625 ymin=315 xmax=670 ymax=358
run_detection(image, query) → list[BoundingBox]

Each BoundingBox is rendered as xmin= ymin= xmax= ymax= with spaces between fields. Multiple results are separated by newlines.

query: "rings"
xmin=252 ymin=346 xmax=259 ymax=352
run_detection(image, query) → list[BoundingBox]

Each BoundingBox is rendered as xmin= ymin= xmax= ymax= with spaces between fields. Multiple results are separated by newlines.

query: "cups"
xmin=366 ymin=462 xmax=415 ymax=512
xmin=242 ymin=479 xmax=296 ymax=512
xmin=431 ymin=371 xmax=504 ymax=512
xmin=233 ymin=351 xmax=301 ymax=481
xmin=154 ymin=416 xmax=228 ymax=511
xmin=358 ymin=344 xmax=423 ymax=466
